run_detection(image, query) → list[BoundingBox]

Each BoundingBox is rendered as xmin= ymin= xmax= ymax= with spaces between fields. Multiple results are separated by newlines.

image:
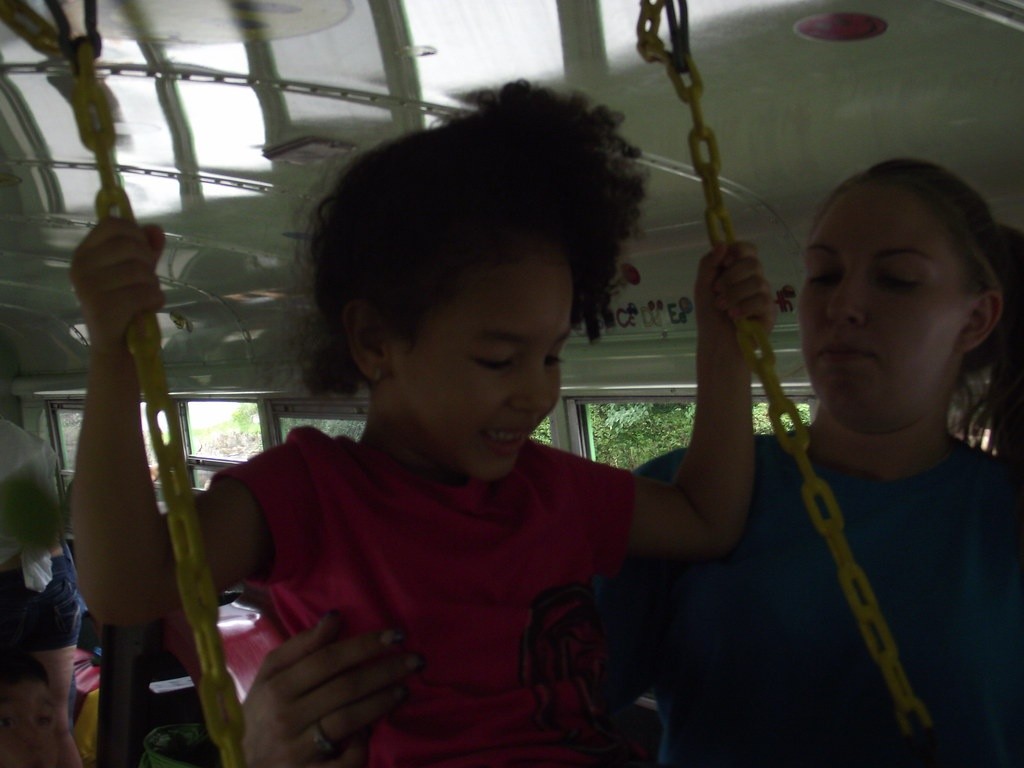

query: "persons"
xmin=0 ymin=458 xmax=87 ymax=768
xmin=240 ymin=157 xmax=1024 ymax=768
xmin=66 ymin=88 xmax=785 ymax=768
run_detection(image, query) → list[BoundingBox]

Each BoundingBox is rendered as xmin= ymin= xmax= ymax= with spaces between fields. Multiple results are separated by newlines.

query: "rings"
xmin=313 ymin=720 xmax=336 ymax=754
